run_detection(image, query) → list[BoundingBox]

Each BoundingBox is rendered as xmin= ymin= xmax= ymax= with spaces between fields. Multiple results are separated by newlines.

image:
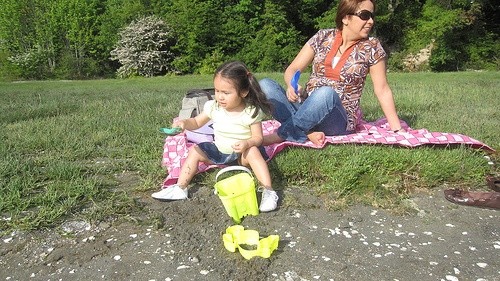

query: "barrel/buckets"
xmin=214 ymin=166 xmax=260 ymax=224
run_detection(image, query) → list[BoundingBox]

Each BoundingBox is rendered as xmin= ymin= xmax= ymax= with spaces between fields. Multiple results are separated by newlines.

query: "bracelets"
xmin=394 ymin=127 xmax=402 ymax=133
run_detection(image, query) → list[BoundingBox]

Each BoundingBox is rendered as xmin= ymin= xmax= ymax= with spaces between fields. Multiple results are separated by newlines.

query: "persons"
xmin=151 ymin=62 xmax=278 ymax=212
xmin=256 ymin=0 xmax=405 ymax=146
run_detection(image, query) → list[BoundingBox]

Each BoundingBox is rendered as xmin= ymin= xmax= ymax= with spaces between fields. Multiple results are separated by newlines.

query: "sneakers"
xmin=151 ymin=184 xmax=188 ymax=200
xmin=258 ymin=186 xmax=278 ymax=212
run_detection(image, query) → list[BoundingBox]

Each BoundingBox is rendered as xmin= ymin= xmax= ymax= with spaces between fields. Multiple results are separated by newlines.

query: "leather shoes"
xmin=444 ymin=188 xmax=500 ymax=209
xmin=486 ymin=176 xmax=500 ymax=193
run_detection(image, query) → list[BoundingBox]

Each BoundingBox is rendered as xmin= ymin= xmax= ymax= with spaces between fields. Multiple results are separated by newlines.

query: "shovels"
xmin=290 ymin=71 xmax=299 ymax=94
xmin=161 ymin=127 xmax=181 ymax=135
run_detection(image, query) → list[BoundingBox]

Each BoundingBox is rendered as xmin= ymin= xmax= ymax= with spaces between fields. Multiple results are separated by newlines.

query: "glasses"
xmin=347 ymin=10 xmax=374 ymax=20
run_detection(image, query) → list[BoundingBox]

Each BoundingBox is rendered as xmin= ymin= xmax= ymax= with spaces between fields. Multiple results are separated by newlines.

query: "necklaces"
xmin=339 ymin=48 xmax=343 ymax=56
